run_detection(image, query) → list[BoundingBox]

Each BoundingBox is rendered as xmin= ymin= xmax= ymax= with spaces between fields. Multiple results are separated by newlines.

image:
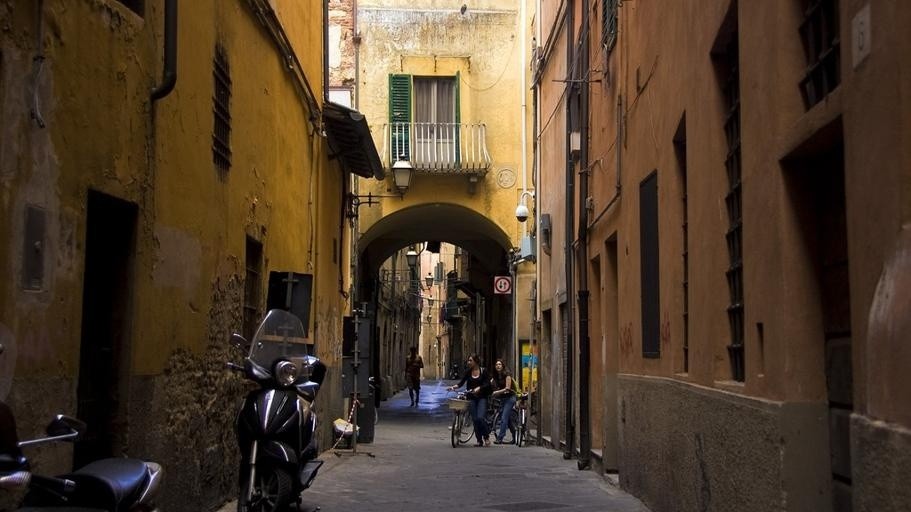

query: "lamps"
xmin=425 ymin=273 xmax=434 ymax=289
xmin=391 ymin=154 xmax=415 ymax=195
xmin=428 ymin=295 xmax=434 ymax=306
xmin=405 ymin=246 xmax=419 ymax=270
xmin=516 ymin=191 xmax=535 ymax=222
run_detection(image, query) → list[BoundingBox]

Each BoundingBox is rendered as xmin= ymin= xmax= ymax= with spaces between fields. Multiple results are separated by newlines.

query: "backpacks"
xmin=510 ymin=377 xmax=522 ymax=400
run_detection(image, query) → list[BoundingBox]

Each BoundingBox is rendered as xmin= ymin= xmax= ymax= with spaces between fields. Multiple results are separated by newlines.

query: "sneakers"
xmin=474 ymin=442 xmax=483 ymax=447
xmin=485 ymin=436 xmax=490 ymax=445
xmin=494 ymin=439 xmax=502 ymax=444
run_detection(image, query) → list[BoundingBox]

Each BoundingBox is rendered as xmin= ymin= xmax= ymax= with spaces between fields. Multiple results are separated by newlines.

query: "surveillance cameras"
xmin=514 ymin=203 xmax=529 ymax=223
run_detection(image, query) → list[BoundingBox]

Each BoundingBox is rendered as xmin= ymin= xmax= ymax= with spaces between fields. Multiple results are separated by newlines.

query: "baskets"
xmin=448 ymin=398 xmax=472 ymax=411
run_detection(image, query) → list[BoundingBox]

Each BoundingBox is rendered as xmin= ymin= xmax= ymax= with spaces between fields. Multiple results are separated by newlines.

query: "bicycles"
xmin=442 ymin=385 xmax=539 ymax=448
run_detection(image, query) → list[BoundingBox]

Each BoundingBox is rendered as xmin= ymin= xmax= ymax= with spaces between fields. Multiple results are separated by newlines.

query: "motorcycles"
xmin=1 ymin=410 xmax=163 ymax=510
xmin=226 ymin=309 xmax=327 ymax=511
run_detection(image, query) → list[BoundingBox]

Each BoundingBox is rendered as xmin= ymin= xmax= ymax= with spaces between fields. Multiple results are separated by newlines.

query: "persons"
xmin=404 ymin=346 xmax=425 ymax=406
xmin=490 ymin=358 xmax=517 ymax=444
xmin=445 ymin=352 xmax=492 ymax=447
